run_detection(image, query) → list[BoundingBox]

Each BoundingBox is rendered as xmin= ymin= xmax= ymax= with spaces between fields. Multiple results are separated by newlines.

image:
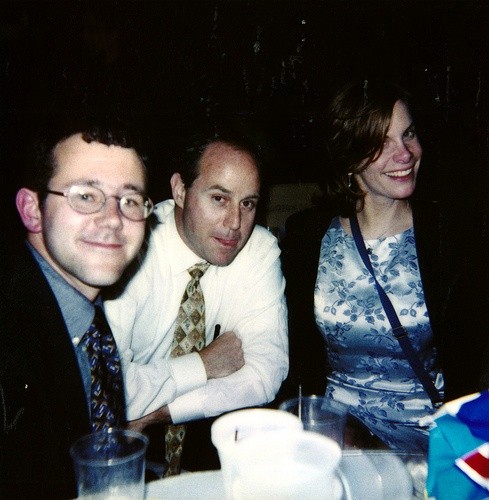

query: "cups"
xmin=279 ymin=396 xmax=349 ymax=451
xmin=210 ymin=408 xmax=304 ymax=500
xmin=70 ymin=429 xmax=149 ymax=500
xmin=232 ymin=430 xmax=343 ymax=500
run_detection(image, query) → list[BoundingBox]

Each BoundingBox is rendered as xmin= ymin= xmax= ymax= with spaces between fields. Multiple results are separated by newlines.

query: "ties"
xmin=163 ymin=263 xmax=211 ymax=476
xmin=76 ymin=305 xmax=136 ymax=491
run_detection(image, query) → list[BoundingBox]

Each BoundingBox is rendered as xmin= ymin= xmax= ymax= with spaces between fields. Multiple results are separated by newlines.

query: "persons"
xmin=1 ymin=111 xmax=157 ymax=500
xmin=104 ymin=133 xmax=290 ymax=438
xmin=279 ymin=79 xmax=488 ymax=461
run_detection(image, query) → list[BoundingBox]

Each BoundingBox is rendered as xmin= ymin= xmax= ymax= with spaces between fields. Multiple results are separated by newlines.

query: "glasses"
xmin=46 ymin=185 xmax=157 ymax=220
